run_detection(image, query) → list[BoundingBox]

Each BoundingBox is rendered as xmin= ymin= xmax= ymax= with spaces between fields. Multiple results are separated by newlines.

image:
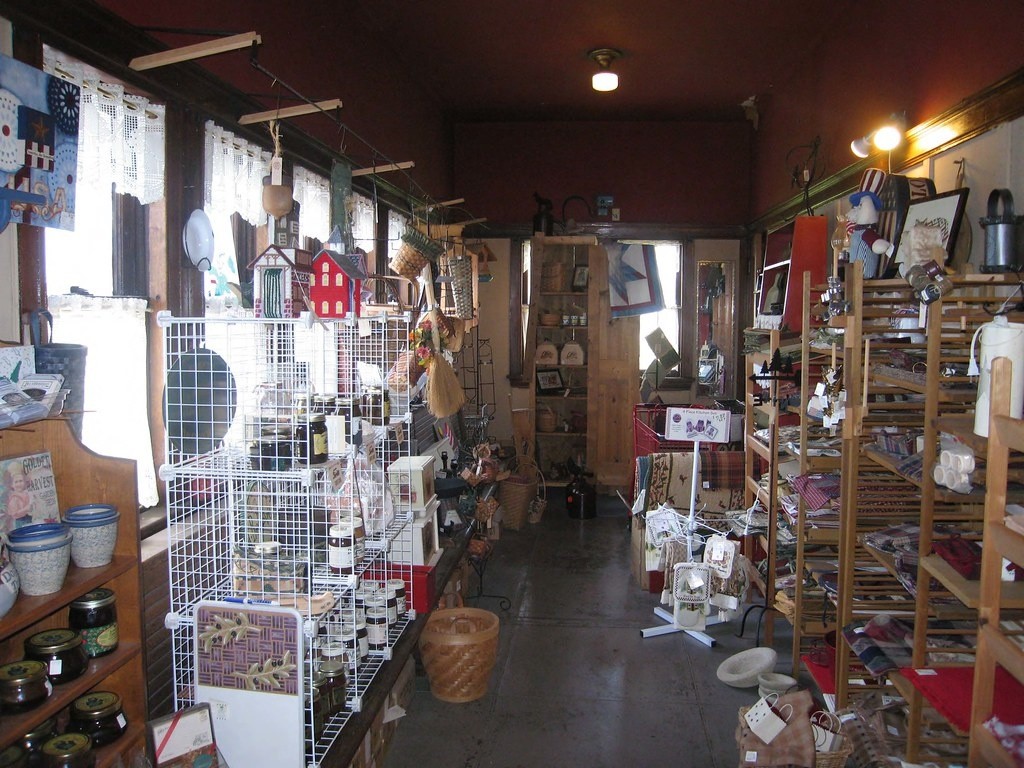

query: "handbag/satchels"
xmin=810 ymin=710 xmax=843 ymax=752
xmin=744 ymin=693 xmax=794 ymax=746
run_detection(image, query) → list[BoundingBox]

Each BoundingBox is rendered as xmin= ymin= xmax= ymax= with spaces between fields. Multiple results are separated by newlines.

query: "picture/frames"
xmin=883 ymin=187 xmax=969 ymax=279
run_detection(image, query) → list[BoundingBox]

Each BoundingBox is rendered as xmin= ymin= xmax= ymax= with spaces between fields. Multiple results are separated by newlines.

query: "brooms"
xmin=425 ymin=263 xmax=467 ymax=419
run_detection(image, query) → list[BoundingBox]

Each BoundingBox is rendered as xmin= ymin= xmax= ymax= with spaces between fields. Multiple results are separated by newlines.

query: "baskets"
xmin=419 ymin=590 xmax=500 ymax=703
xmin=386 ymin=351 xmax=426 ymax=393
xmin=498 ymin=454 xmax=546 ymax=531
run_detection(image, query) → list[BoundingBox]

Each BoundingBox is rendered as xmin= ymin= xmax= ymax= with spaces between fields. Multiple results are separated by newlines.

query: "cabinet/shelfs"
xmin=508 ymin=236 xmax=640 ymax=498
xmin=0 ymin=412 xmax=153 ymax=768
xmin=743 ymin=216 xmax=1024 ymax=768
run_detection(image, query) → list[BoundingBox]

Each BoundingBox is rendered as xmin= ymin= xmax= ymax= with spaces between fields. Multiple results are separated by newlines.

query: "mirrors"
xmin=695 ymin=260 xmax=735 ymax=401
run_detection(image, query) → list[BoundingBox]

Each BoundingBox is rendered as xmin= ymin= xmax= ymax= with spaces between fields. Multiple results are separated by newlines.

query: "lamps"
xmin=588 ymin=49 xmax=621 ymax=92
xmin=850 ymin=110 xmax=907 ymax=158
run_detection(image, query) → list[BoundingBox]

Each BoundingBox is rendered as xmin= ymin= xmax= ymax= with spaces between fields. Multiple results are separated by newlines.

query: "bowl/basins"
xmin=758 ymin=673 xmax=797 ymax=701
xmin=540 ymin=314 xmax=560 ymax=325
xmin=182 ymin=209 xmax=215 ymax=272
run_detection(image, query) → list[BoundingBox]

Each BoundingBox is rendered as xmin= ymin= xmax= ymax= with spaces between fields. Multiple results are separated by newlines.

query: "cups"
xmin=563 ymin=315 xmax=587 ymax=325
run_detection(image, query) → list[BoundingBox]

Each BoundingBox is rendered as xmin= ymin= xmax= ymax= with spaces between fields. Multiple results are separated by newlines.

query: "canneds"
xmin=0 ymin=588 xmax=128 ymax=768
xmin=253 ymin=387 xmax=405 ymax=745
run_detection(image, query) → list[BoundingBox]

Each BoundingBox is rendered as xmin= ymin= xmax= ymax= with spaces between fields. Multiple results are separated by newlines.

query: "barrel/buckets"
xmin=980 ymin=187 xmax=1024 ymax=272
